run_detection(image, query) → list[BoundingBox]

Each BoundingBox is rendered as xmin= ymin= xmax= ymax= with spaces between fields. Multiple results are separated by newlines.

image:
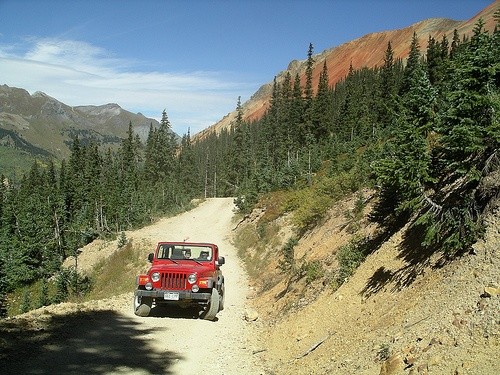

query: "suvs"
xmin=133 ymin=240 xmax=226 ymax=321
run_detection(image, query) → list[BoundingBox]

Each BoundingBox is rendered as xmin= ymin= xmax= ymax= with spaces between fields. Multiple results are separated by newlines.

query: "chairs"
xmin=173 ymin=247 xmax=192 ymax=260
xmin=199 ymin=250 xmax=211 ymax=260
